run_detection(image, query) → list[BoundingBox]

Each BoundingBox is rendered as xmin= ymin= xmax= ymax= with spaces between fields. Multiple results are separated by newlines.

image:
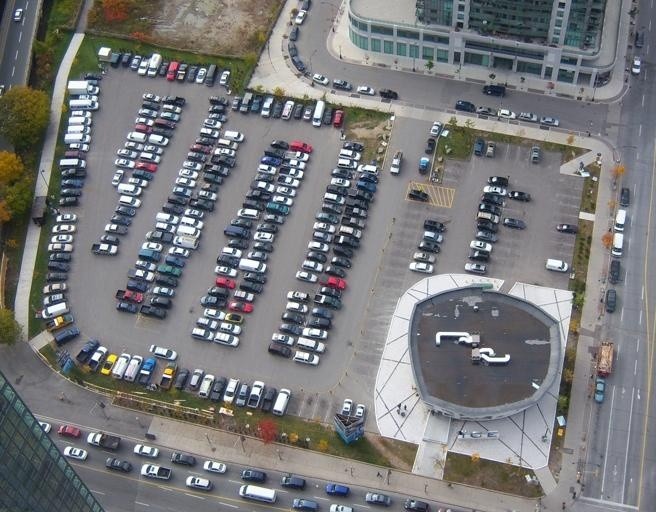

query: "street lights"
xmin=89 ymin=37 xmax=99 ymax=63
xmin=320 ymin=1 xmax=335 ymax=33
xmin=576 ymin=461 xmax=602 ymax=468
xmin=612 ymin=145 xmax=638 ymax=162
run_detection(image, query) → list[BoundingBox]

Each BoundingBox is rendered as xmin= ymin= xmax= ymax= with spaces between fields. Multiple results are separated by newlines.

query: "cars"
xmin=287 ymin=0 xmax=312 ymax=55
xmin=409 ymin=101 xmax=579 ymax=276
xmin=0 ymin=85 xmax=5 ymax=97
xmin=631 ymin=56 xmax=641 ymax=76
xmin=604 ymin=187 xmax=631 ymax=312
xmin=636 ymin=29 xmax=645 ymax=48
xmin=595 ymin=378 xmax=606 ymax=402
xmin=13 ymin=8 xmax=23 ymax=22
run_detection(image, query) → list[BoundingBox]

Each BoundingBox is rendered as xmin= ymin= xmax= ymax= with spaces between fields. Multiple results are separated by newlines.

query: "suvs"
xmin=381 ymin=88 xmax=397 ymax=100
xmin=483 ymin=85 xmax=506 ymax=97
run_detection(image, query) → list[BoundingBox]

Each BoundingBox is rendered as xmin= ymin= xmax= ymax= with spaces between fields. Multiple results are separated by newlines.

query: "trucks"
xmin=598 ymin=342 xmax=615 ymax=377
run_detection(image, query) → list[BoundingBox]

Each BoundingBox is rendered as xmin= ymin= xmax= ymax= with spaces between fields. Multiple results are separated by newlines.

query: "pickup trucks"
xmin=390 ymin=151 xmax=403 ymax=176
